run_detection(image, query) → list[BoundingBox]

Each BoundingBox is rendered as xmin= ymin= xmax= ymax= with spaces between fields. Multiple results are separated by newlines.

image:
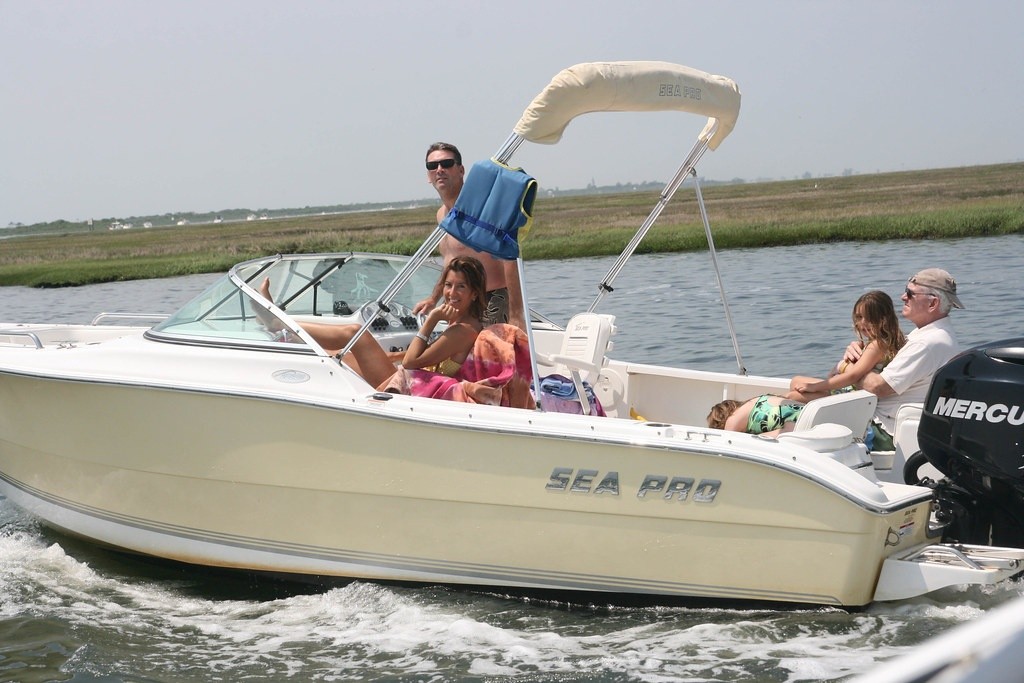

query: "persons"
xmin=705 ymin=394 xmax=806 ymax=437
xmin=251 ymin=257 xmax=487 ymax=392
xmin=828 ymin=267 xmax=964 ymax=451
xmin=789 ymin=291 xmax=906 ymax=404
xmin=412 ymin=143 xmax=525 ymax=333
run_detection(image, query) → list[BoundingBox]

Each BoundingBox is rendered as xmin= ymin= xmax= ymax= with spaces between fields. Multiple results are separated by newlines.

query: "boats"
xmin=0 ymin=60 xmax=1024 ymax=618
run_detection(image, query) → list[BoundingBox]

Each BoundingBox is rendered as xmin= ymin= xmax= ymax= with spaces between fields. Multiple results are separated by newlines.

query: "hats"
xmin=909 ymin=268 xmax=966 ymax=309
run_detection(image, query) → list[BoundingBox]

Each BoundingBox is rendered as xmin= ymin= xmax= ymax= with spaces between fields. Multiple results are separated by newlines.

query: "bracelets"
xmin=842 ymin=362 xmax=850 ymax=373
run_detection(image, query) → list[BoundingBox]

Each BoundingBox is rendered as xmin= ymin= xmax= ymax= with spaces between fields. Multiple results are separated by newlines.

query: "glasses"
xmin=426 ymin=158 xmax=460 ymax=170
xmin=904 ymin=285 xmax=936 ymax=299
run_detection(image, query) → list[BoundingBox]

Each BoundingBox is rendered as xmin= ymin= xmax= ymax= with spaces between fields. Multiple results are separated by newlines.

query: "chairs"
xmin=776 ymin=388 xmax=880 ymax=453
xmin=867 ymin=400 xmax=928 ymax=470
xmin=393 ymin=321 xmax=531 ymax=408
xmin=532 ymin=312 xmax=617 ymax=404
